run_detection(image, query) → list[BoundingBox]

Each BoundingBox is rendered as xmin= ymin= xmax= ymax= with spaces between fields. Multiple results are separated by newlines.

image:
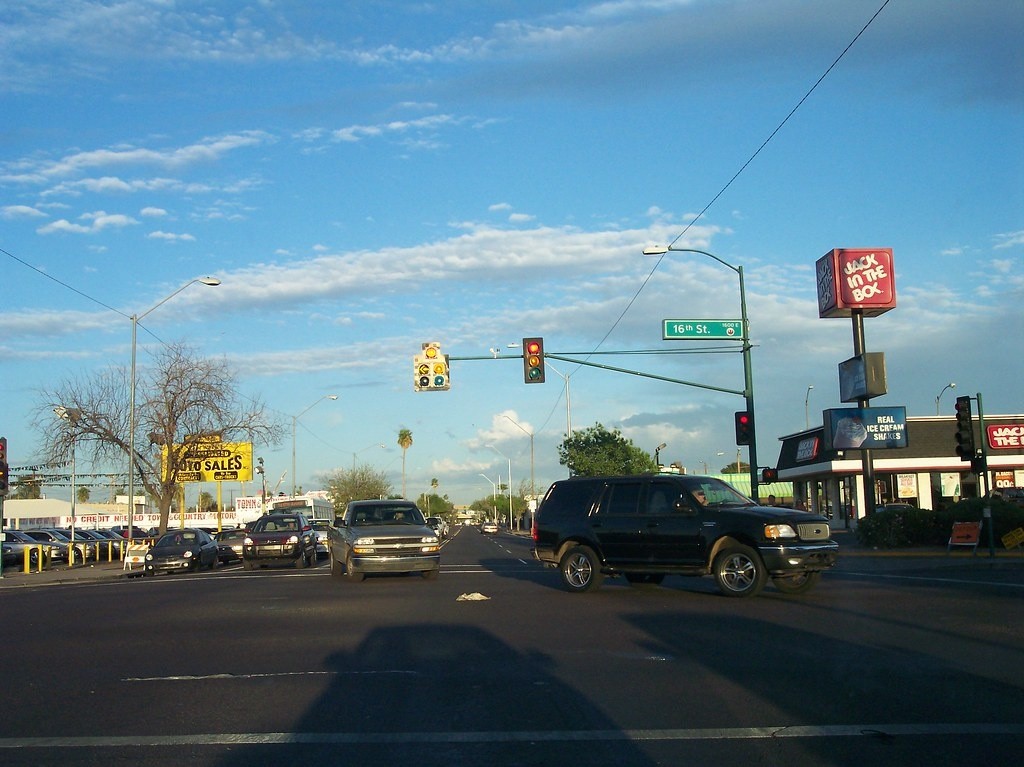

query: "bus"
xmin=267 ymin=498 xmax=337 ymax=555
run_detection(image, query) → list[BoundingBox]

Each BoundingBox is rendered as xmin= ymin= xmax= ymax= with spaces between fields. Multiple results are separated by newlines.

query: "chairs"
xmin=651 ymin=490 xmax=669 ymax=512
xmin=267 ymin=522 xmax=276 ymax=529
xmin=355 ymin=512 xmax=367 ymax=522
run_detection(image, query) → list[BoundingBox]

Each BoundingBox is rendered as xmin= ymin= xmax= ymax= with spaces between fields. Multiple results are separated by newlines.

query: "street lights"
xmin=499 ymin=414 xmax=536 ymax=537
xmin=506 ymin=342 xmax=574 ymax=477
xmin=642 ymin=245 xmax=762 ymax=504
xmin=478 ymin=473 xmax=496 ymax=523
xmin=291 ymin=394 xmax=339 ymax=498
xmin=352 ymin=440 xmax=387 ymax=477
xmin=804 ymin=385 xmax=814 ymax=434
xmin=655 ymin=442 xmax=667 ymax=468
xmin=127 ymin=275 xmax=222 ymax=543
xmin=252 ymin=458 xmax=266 ymax=514
xmin=424 ymin=485 xmax=438 ymax=516
xmin=485 ymin=443 xmax=513 ymax=533
xmin=937 ymin=383 xmax=956 ymax=417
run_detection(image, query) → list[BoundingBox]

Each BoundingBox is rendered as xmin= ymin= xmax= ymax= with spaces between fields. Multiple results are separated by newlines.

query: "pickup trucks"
xmin=326 ymin=499 xmax=444 ymax=580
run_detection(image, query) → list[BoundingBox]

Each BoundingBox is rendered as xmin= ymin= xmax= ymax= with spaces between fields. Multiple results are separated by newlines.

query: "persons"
xmin=288 ymin=523 xmax=295 ymax=528
xmin=236 ymin=523 xmax=241 ymax=528
xmin=171 ymin=534 xmax=185 ymax=544
xmin=769 ymin=495 xmax=778 ymax=506
xmin=795 ymin=499 xmax=806 ymax=510
xmin=394 ymin=511 xmax=403 ymax=519
xmin=689 ymin=484 xmax=709 ymax=507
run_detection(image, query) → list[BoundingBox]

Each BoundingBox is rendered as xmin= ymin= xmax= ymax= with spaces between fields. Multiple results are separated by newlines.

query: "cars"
xmin=2 ymin=524 xmax=178 ymax=566
xmin=144 ymin=529 xmax=219 ymax=575
xmin=877 ymin=502 xmax=918 ymax=511
xmin=981 ymin=486 xmax=1024 ymax=505
xmin=481 ymin=522 xmax=498 ymax=533
xmin=430 ymin=516 xmax=481 ymax=538
xmin=215 ymin=528 xmax=247 ymax=564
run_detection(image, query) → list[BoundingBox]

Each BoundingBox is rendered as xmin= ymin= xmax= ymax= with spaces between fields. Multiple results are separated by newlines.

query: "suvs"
xmin=534 ymin=475 xmax=840 ymax=597
xmin=242 ymin=513 xmax=319 ymax=570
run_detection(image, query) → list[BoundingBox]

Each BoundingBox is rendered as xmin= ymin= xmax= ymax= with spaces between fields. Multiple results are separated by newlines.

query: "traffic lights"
xmin=971 ymin=457 xmax=984 ymax=473
xmin=0 ymin=464 xmax=8 ymax=496
xmin=421 ymin=341 xmax=443 ymax=361
xmin=954 ymin=395 xmax=974 ymax=461
xmin=523 ymin=337 xmax=545 ymax=384
xmin=734 ymin=411 xmax=753 ymax=446
xmin=414 ymin=362 xmax=434 ymax=393
xmin=431 ymin=360 xmax=450 ymax=391
xmin=0 ymin=437 xmax=7 ymax=465
xmin=763 ymin=469 xmax=778 ymax=482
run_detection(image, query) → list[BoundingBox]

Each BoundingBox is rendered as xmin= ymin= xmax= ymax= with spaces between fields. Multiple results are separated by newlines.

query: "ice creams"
xmin=833 ymin=416 xmax=867 ymax=448
